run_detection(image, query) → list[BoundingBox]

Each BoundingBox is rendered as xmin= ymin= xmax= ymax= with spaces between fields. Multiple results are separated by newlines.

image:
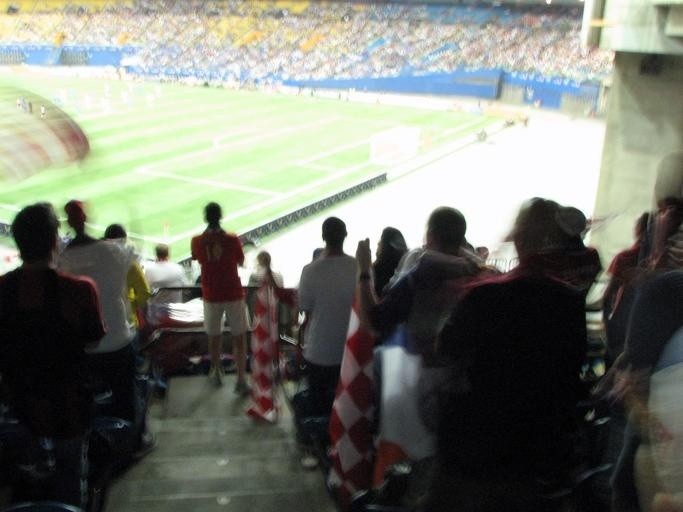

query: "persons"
xmin=0 ymin=196 xmax=182 ymax=457
xmin=291 ymin=152 xmax=683 ymax=512
xmin=190 ymin=202 xmax=283 ymax=396
xmin=0 ymin=0 xmax=615 ymax=122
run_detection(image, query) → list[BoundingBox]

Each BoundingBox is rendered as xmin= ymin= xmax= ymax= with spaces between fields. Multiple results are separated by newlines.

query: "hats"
xmin=504 ymin=197 xmax=562 ymax=242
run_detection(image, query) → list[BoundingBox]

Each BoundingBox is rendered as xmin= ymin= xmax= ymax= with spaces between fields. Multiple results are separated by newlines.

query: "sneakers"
xmin=134 ymin=433 xmax=155 ymax=459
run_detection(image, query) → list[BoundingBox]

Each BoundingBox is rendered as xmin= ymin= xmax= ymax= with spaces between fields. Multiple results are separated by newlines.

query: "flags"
xmin=244 ymin=274 xmax=280 ymax=425
